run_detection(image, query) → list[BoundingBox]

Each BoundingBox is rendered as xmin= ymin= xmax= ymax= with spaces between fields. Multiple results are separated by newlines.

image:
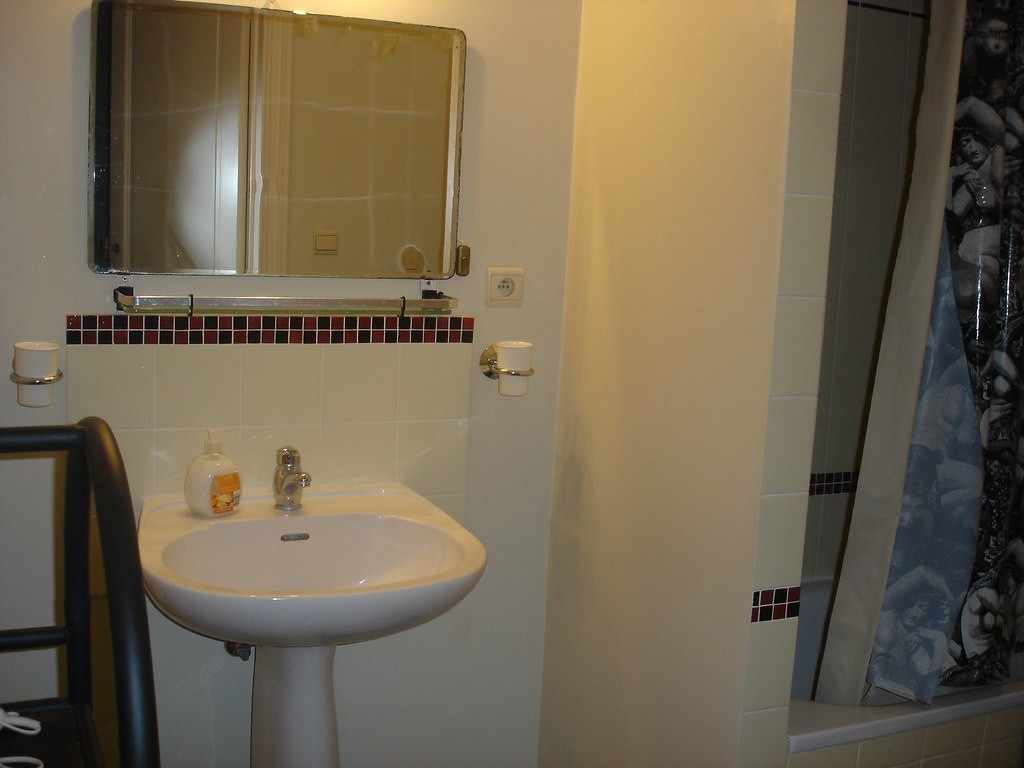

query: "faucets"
xmin=272 ymin=447 xmax=313 ymax=510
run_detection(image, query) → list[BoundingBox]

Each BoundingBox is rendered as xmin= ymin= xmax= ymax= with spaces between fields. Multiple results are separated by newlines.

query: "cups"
xmin=14 ymin=341 xmax=60 ymax=407
xmin=497 ymin=341 xmax=533 ymax=396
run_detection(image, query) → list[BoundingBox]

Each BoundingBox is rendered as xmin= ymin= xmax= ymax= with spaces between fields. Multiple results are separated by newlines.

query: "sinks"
xmin=136 ymin=480 xmax=487 ymax=650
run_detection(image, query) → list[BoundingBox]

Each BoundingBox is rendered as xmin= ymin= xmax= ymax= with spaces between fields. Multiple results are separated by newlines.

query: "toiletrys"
xmin=184 ymin=426 xmax=244 ymax=517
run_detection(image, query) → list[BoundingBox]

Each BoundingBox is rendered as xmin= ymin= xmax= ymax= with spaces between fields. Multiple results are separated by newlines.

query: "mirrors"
xmin=87 ymin=1 xmax=467 ymax=282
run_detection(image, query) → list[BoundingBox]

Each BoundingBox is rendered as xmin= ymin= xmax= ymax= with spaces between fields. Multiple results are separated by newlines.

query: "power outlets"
xmin=485 ymin=269 xmax=526 ymax=309
